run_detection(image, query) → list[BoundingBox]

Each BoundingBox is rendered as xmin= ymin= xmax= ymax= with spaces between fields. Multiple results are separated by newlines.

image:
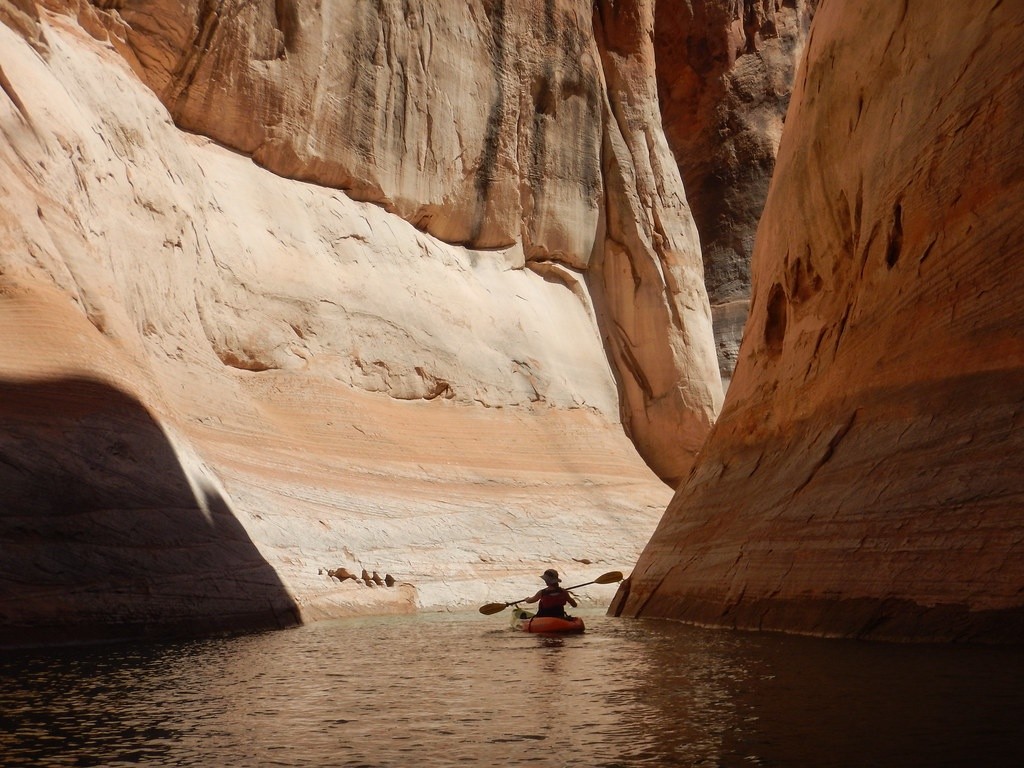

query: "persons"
xmin=525 ymin=569 xmax=579 ymax=617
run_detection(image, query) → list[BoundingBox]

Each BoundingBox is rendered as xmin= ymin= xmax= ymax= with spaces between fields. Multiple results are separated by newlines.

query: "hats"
xmin=540 ymin=569 xmax=563 ymax=583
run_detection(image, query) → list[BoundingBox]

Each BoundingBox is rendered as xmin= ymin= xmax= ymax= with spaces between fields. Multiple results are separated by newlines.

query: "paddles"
xmin=479 ymin=571 xmax=623 ymax=616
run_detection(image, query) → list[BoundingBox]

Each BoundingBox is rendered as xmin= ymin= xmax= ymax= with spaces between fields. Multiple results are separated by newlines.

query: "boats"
xmin=510 ymin=607 xmax=587 ymax=634
